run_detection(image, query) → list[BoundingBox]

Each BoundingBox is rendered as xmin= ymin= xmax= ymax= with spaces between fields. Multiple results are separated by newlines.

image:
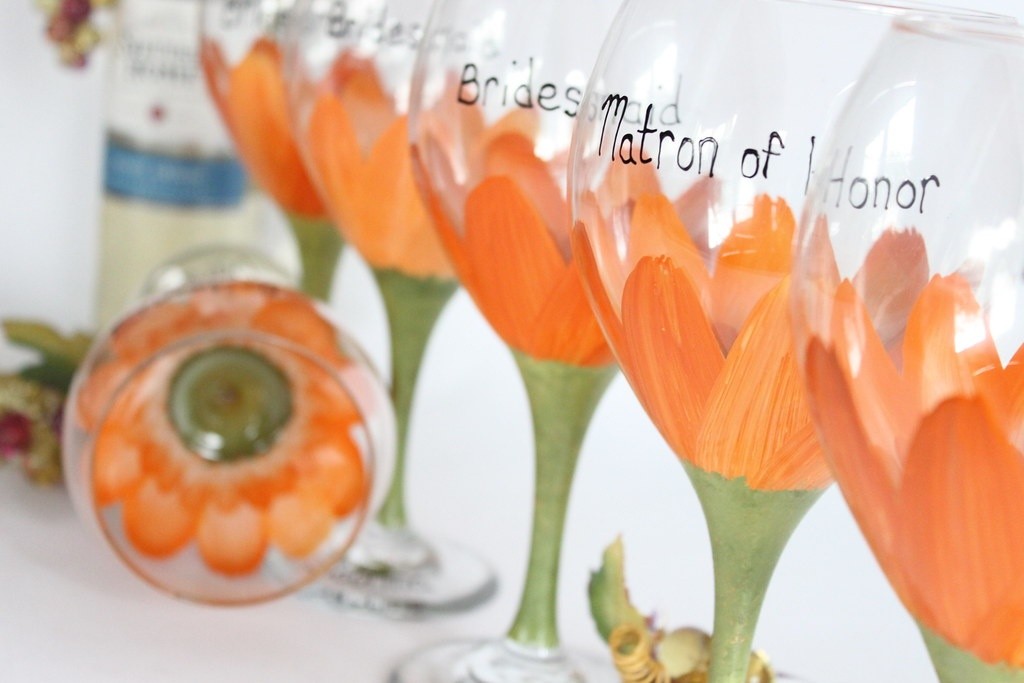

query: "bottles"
xmin=94 ymin=0 xmax=253 ymax=326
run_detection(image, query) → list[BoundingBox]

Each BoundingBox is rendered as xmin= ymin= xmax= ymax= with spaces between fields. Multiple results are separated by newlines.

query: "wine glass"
xmin=782 ymin=10 xmax=1024 ymax=683
xmin=566 ymin=2 xmax=1013 ymax=683
xmin=281 ymin=1 xmax=502 ymax=621
xmin=60 ymin=239 xmax=398 ymax=610
xmin=392 ymin=1 xmax=616 ymax=683
xmin=193 ymin=0 xmax=353 ymax=306
